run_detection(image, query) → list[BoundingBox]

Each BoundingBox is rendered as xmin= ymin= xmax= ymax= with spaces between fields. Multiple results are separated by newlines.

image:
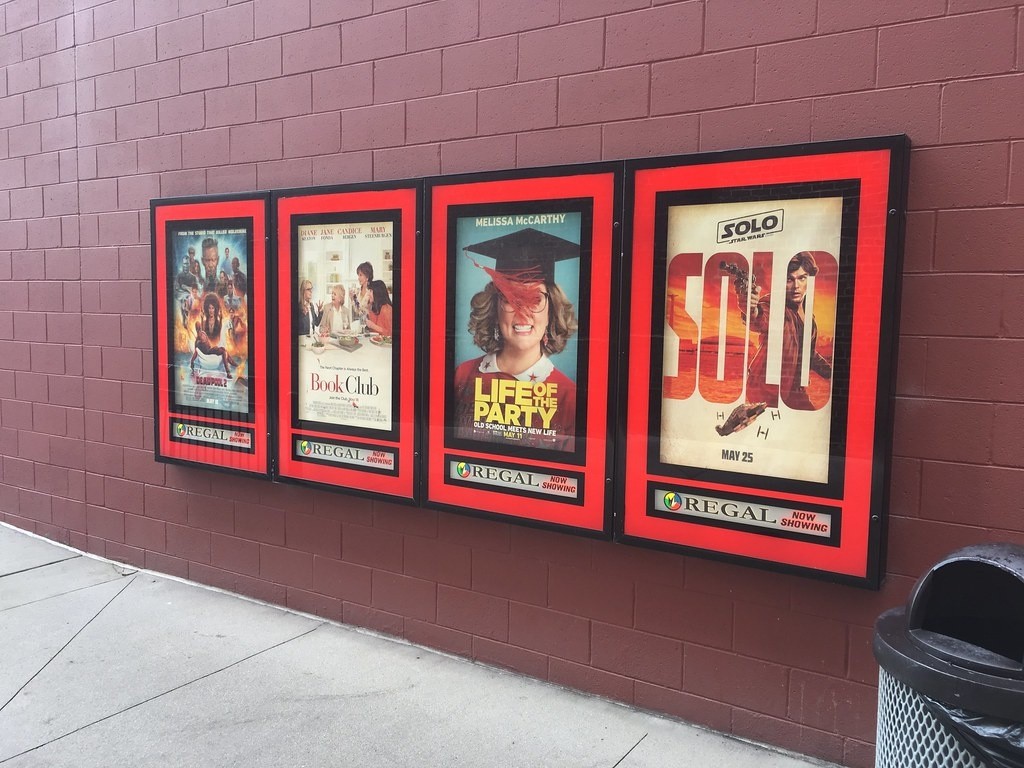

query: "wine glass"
xmin=343 ymin=323 xmax=348 ymax=330
xmin=360 ymin=317 xmax=367 ymax=334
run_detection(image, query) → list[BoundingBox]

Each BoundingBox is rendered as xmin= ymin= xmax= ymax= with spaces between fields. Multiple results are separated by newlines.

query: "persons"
xmin=298 ymin=261 xmax=392 ymax=335
xmin=454 ymin=228 xmax=580 ymax=452
xmin=735 ymin=252 xmax=831 ymax=411
xmin=175 ymin=238 xmax=247 ymax=379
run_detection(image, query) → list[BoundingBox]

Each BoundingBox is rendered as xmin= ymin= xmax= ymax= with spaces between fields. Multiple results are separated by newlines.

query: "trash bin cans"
xmin=871 ymin=539 xmax=1024 ymax=768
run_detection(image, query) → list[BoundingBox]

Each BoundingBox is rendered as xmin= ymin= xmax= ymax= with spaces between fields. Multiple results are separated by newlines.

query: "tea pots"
xmin=340 ymin=335 xmax=355 ymax=346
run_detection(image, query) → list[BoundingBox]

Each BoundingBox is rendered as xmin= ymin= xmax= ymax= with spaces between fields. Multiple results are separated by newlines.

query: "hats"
xmin=462 ymin=227 xmax=580 ymax=326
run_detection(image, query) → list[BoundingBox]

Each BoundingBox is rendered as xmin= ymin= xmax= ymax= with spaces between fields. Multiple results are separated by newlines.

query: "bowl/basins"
xmin=312 ymin=342 xmax=325 ymax=353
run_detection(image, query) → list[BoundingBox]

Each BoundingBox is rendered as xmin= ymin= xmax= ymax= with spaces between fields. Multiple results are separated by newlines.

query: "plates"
xmin=369 ymin=336 xmax=392 ymax=346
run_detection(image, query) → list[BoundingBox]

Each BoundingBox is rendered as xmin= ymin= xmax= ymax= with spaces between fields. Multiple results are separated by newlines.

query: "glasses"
xmin=307 ymin=288 xmax=312 ymax=291
xmin=500 ymin=292 xmax=550 ymax=313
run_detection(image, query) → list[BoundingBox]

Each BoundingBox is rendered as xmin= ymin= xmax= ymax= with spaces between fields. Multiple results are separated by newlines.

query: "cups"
xmin=299 ymin=335 xmax=307 ymax=345
xmin=318 ymin=336 xmax=329 ymax=345
